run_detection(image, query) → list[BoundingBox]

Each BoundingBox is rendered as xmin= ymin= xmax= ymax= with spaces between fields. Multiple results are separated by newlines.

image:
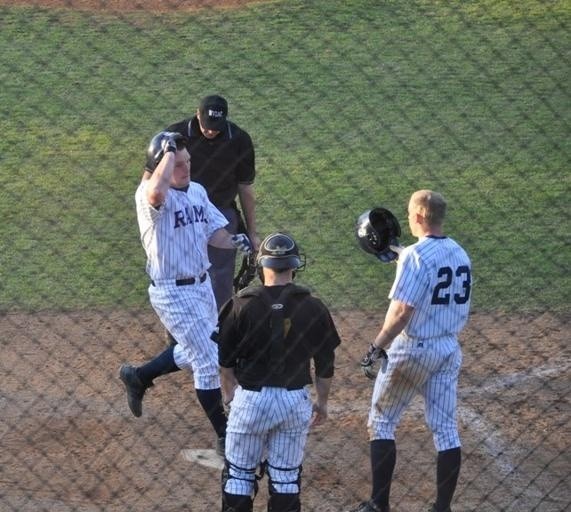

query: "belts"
xmin=151 ymin=273 xmax=206 ymax=286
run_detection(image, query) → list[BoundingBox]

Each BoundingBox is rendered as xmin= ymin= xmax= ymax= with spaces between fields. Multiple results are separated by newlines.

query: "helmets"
xmin=355 ymin=207 xmax=401 ymax=261
xmin=146 ymin=131 xmax=183 ymax=170
xmin=256 ymin=233 xmax=300 ymax=270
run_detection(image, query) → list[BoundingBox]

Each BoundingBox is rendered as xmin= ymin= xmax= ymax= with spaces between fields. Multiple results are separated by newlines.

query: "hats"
xmin=199 ymin=95 xmax=227 ymax=131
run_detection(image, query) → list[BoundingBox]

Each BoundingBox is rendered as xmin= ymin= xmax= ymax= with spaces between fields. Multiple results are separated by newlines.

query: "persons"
xmin=162 ymin=95 xmax=261 ymax=373
xmin=117 ymin=129 xmax=253 ymax=459
xmin=356 ymin=188 xmax=478 ymax=510
xmin=215 ymin=231 xmax=339 ymax=511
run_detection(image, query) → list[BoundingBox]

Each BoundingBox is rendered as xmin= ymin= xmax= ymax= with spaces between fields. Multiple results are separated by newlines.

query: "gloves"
xmin=360 ymin=344 xmax=388 ymax=380
xmin=232 ymin=234 xmax=253 ymax=255
xmin=161 ymin=136 xmax=177 ymax=154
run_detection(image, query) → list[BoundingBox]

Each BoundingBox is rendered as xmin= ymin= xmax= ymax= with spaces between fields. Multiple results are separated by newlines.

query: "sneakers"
xmin=119 ymin=366 xmax=153 ymax=417
xmin=349 ymin=501 xmax=389 ymax=512
xmin=216 ymin=437 xmax=225 ymax=457
